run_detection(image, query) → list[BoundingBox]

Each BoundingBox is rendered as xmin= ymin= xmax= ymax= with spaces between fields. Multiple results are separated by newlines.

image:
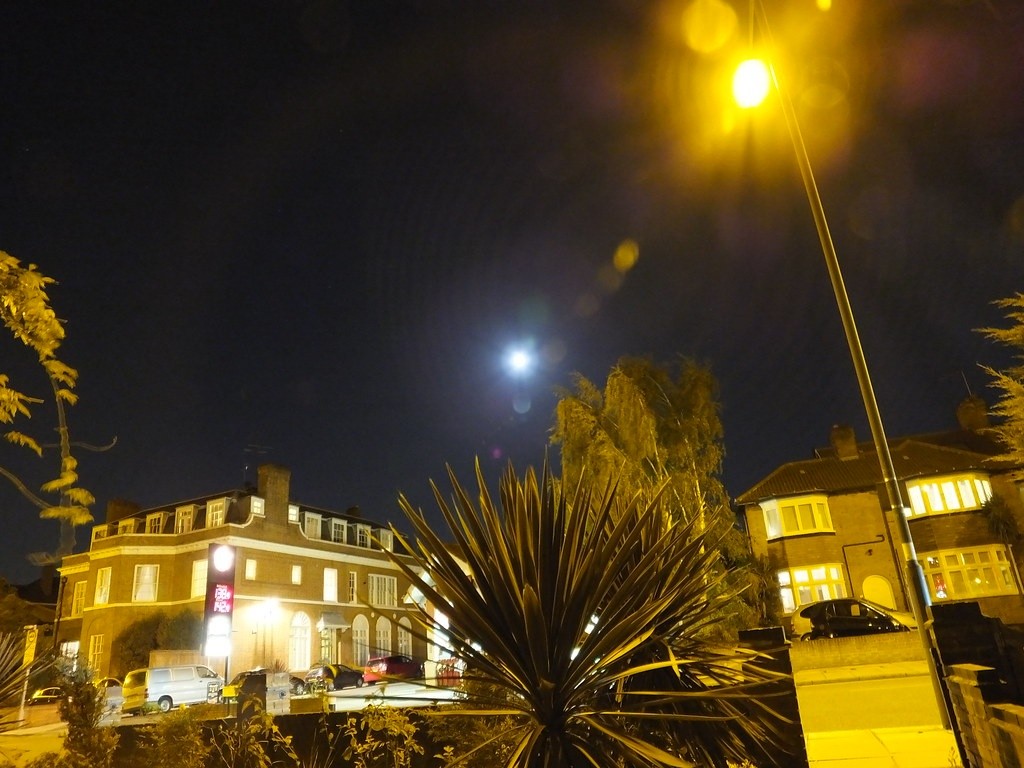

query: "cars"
xmin=304 ymin=664 xmax=363 ymax=692
xmin=27 ymin=686 xmax=66 ymax=706
xmin=94 ymin=676 xmax=124 ymax=698
xmin=790 ymin=596 xmax=918 ymax=642
xmin=363 ymin=656 xmax=423 ymax=686
xmin=222 ymin=668 xmax=305 ymax=701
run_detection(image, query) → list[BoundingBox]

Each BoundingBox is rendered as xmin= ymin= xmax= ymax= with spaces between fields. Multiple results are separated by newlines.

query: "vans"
xmin=120 ymin=664 xmax=231 ymax=716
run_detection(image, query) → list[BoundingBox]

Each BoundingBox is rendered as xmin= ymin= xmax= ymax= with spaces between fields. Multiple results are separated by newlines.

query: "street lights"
xmin=51 ymin=575 xmax=69 ymax=661
xmin=730 ymin=1 xmax=957 ymax=732
